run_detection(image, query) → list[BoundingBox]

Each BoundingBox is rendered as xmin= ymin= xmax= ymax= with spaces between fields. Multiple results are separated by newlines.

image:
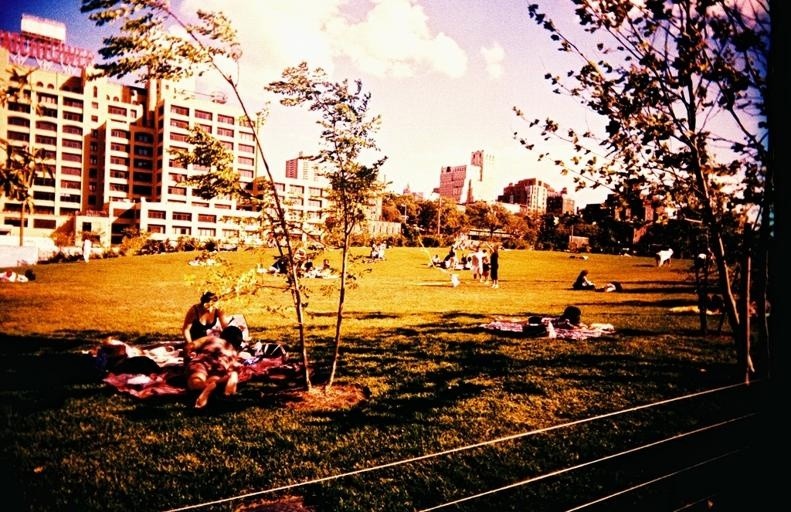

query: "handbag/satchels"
xmin=524 ymin=322 xmax=555 ymax=338
xmin=255 ymin=343 xmax=286 ymax=358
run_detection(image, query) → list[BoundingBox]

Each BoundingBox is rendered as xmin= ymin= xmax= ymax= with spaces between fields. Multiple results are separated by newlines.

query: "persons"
xmin=81 ymin=234 xmax=93 ymax=264
xmin=366 ymin=238 xmax=390 ymax=262
xmin=574 ymin=270 xmax=597 ymax=292
xmin=182 ymin=291 xmax=228 ymax=365
xmin=426 ymin=238 xmax=506 ymax=290
xmin=590 ymin=279 xmax=624 ymax=294
xmin=182 ymin=326 xmax=244 ymax=409
xmin=485 ymin=306 xmax=596 ymax=332
xmin=134 ymin=236 xmax=239 ymax=268
xmin=255 ymin=235 xmax=342 ymax=281
xmin=0 ymin=267 xmax=31 ymax=284
